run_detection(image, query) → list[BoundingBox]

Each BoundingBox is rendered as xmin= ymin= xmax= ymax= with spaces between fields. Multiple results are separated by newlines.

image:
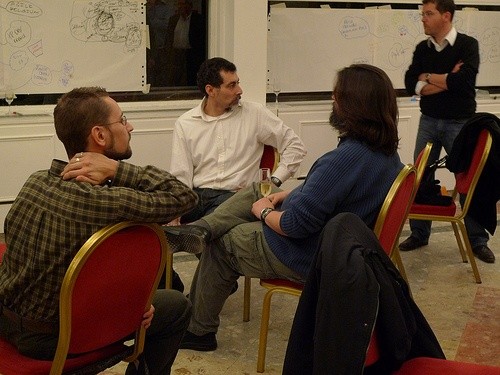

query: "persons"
xmin=163 ymin=63 xmax=402 ymax=352
xmin=398 ymin=0 xmax=496 ymax=264
xmin=0 ymin=86 xmax=198 ymax=374
xmin=165 ymin=0 xmax=204 ymax=86
xmin=160 ymin=57 xmax=307 ymax=293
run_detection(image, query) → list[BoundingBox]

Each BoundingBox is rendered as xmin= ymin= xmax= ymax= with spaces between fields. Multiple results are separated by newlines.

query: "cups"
xmin=259 ymin=168 xmax=271 ymax=198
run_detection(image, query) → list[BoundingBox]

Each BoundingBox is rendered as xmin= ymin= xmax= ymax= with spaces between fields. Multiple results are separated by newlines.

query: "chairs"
xmin=0 ymin=124 xmax=500 ymax=375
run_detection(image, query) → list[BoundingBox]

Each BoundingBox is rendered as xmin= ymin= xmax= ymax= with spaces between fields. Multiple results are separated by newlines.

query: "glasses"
xmin=88 ymin=113 xmax=127 ymax=135
xmin=418 ymin=9 xmax=444 ymax=18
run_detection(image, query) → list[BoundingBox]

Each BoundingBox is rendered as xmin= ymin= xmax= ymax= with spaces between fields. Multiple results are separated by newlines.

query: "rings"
xmin=81 ymin=152 xmax=83 ymax=156
xmin=76 ymin=158 xmax=79 ymax=162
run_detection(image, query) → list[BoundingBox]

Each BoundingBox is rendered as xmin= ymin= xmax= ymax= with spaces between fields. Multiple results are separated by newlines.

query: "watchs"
xmin=271 ymin=177 xmax=282 ymax=187
xmin=426 ymin=73 xmax=431 ymax=84
xmin=260 ymin=208 xmax=273 ymax=224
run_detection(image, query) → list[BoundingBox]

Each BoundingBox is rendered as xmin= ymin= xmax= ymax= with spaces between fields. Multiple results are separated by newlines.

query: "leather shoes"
xmin=398 ymin=235 xmax=429 ymax=251
xmin=161 ymin=225 xmax=211 ymax=254
xmin=471 ymin=245 xmax=495 ymax=263
xmin=178 ymin=329 xmax=218 ymax=351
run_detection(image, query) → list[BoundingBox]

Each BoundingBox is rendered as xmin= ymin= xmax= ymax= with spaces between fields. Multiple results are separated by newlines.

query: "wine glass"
xmin=273 ymin=80 xmax=281 ymax=103
